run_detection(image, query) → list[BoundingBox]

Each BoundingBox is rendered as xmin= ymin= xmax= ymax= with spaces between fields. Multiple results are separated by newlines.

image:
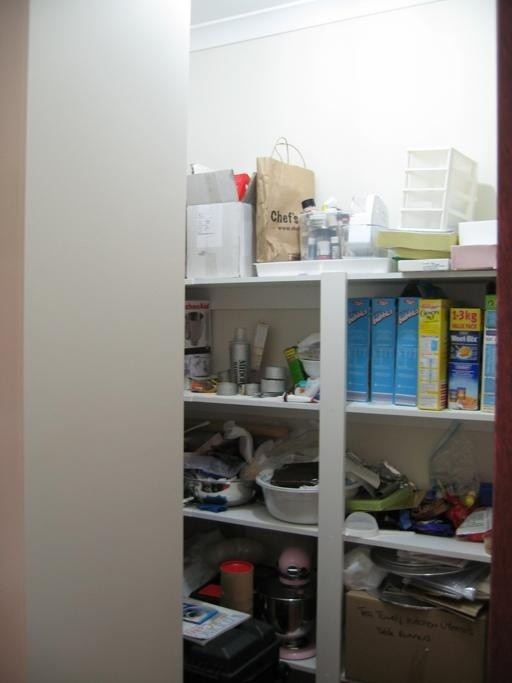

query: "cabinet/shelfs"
xmin=399 ymin=147 xmax=478 ymax=234
xmin=184 ymin=270 xmax=498 ymax=683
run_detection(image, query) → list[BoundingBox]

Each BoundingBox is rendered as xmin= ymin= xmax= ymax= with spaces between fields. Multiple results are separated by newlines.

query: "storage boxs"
xmin=185 ymin=170 xmax=257 ymax=280
xmin=345 ymin=588 xmax=486 ymax=683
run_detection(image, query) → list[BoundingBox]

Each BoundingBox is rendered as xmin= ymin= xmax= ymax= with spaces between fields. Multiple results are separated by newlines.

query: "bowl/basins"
xmin=256 ymin=468 xmax=362 ymax=524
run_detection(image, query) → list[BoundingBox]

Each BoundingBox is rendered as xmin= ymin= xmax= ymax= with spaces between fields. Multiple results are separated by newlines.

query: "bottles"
xmin=301 ymin=197 xmax=350 ymax=258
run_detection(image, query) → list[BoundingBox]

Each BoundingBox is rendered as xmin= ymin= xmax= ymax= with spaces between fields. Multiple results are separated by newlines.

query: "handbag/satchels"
xmin=253 ymin=136 xmax=315 ymax=263
